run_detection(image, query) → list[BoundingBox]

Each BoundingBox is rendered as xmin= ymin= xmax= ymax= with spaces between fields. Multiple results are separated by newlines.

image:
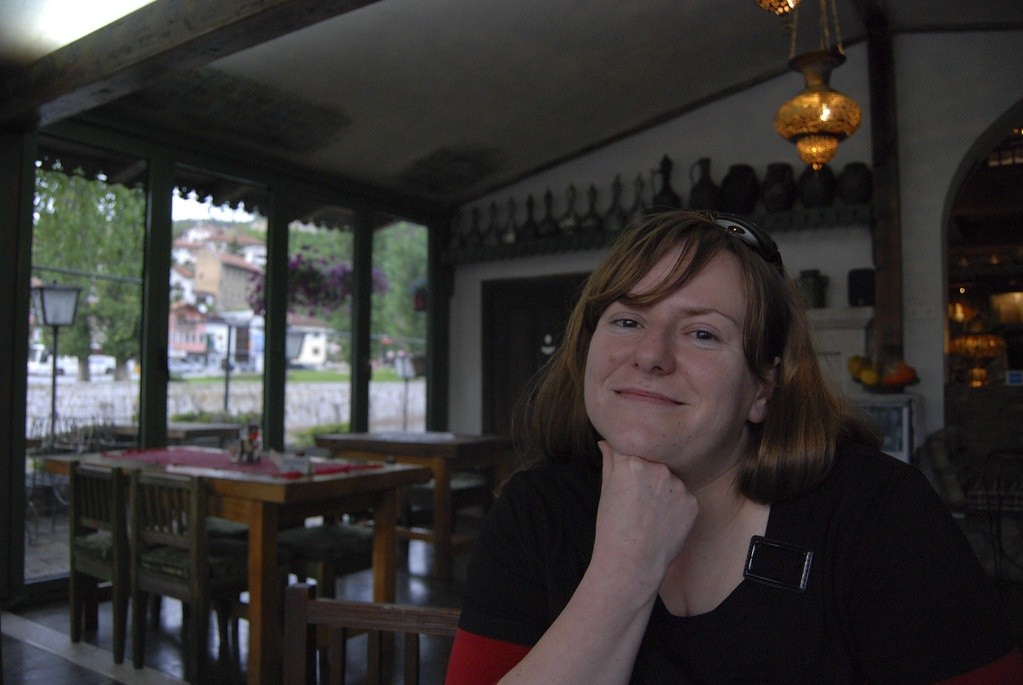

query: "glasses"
xmin=702 ymin=212 xmax=783 ymax=275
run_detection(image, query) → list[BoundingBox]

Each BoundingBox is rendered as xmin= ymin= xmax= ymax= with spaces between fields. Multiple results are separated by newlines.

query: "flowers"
xmin=245 ymin=247 xmax=390 ymax=307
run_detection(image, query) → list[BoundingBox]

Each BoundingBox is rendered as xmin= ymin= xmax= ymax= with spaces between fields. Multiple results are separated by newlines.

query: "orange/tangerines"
xmin=844 ymin=353 xmax=881 ymax=384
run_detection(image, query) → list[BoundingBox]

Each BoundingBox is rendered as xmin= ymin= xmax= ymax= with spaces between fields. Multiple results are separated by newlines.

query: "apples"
xmin=882 ymin=359 xmax=917 ymax=384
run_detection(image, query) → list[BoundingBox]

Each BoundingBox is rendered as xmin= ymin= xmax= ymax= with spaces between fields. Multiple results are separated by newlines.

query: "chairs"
xmin=60 ymin=457 xmax=461 ymax=685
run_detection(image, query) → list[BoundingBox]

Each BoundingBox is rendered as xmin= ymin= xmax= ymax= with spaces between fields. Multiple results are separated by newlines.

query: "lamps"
xmin=755 ymin=0 xmax=800 ymax=15
xmin=773 ymin=0 xmax=862 ymax=170
xmin=951 ymin=258 xmax=1005 ymax=389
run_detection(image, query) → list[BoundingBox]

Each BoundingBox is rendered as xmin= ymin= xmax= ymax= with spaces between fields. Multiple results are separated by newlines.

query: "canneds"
xmin=238 ymin=422 xmax=263 ymax=463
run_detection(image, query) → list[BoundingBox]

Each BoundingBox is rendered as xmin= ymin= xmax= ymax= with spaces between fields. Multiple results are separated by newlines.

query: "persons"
xmin=444 ymin=209 xmax=1023 ymax=685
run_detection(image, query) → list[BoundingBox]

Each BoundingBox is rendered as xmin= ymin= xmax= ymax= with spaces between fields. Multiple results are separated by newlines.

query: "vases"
xmin=293 ymin=284 xmax=339 ymax=310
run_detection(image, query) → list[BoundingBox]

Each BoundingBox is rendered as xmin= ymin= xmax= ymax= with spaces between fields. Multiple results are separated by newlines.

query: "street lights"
xmin=29 ymin=279 xmax=85 ymax=451
xmin=395 ymin=355 xmax=416 ymax=431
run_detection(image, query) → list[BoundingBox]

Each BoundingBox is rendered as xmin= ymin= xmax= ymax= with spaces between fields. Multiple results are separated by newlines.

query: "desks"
xmin=33 ymin=442 xmax=432 ymax=685
xmin=317 ymin=429 xmax=513 ymax=609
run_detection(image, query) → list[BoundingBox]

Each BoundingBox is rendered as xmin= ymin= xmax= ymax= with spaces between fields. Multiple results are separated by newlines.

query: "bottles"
xmin=795 ymin=269 xmax=828 ymax=309
xmin=847 ymin=268 xmax=876 ymax=307
xmin=441 ymin=153 xmax=875 ymax=258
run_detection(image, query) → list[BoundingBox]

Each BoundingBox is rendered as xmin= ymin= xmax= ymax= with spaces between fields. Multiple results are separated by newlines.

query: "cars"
xmin=28 ymin=343 xmax=202 ymax=377
